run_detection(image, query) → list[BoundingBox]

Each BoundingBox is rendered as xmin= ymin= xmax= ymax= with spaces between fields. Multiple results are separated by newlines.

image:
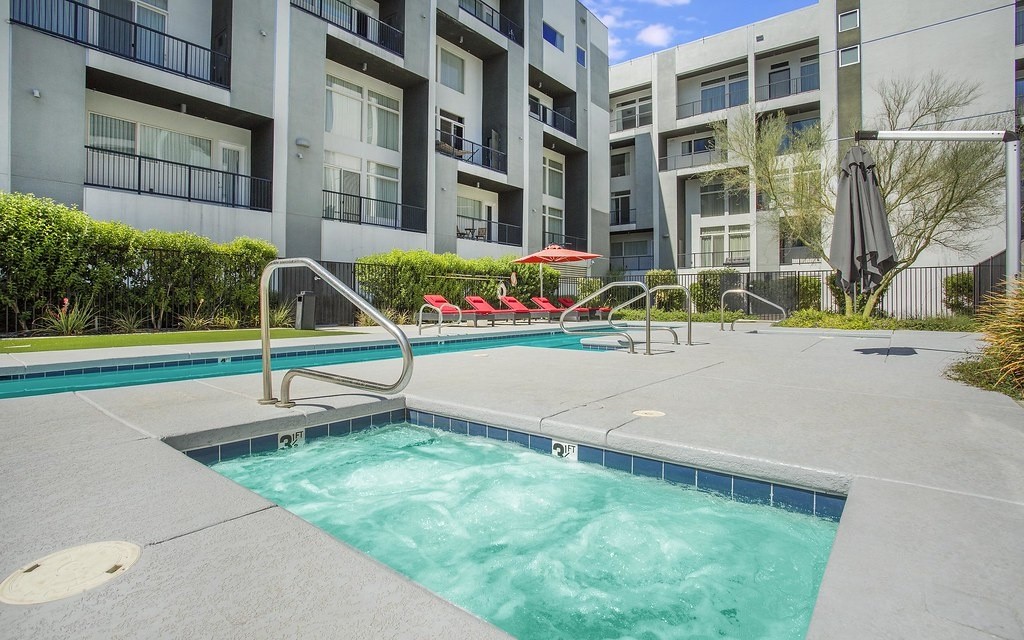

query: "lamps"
xmin=296 ymin=138 xmax=310 ymax=148
xmin=181 ymin=104 xmax=186 ymax=113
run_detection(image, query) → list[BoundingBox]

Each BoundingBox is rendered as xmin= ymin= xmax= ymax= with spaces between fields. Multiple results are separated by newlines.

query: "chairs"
xmin=475 ymin=228 xmax=486 ymax=242
xmin=457 ymin=225 xmax=466 ymax=239
xmin=416 ymin=295 xmax=612 ymax=328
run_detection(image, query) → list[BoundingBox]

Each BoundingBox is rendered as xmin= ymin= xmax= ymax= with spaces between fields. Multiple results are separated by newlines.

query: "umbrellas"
xmin=511 ymin=244 xmax=604 ymax=308
xmin=829 ymin=141 xmax=901 ymax=295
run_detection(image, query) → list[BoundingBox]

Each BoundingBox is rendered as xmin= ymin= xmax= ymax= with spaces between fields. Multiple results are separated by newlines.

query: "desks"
xmin=465 ymin=229 xmax=477 ymax=240
